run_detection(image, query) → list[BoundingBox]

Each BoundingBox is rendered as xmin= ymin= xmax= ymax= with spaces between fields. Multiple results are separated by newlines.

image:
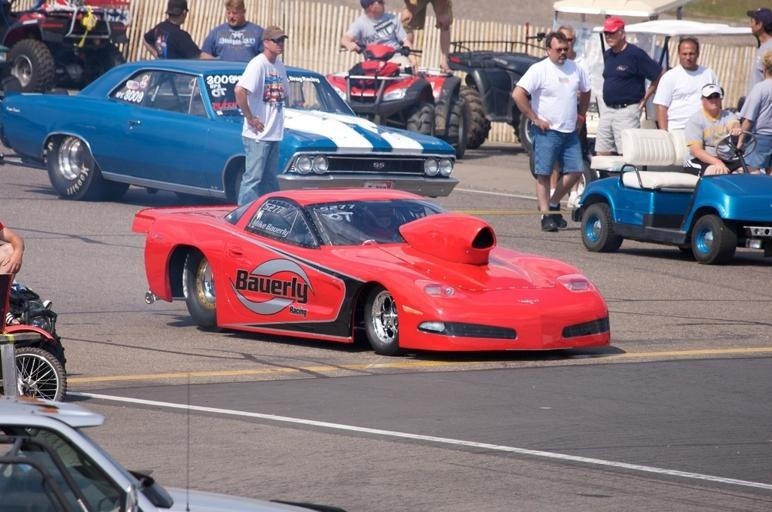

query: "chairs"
xmin=669 ymin=128 xmax=700 ymax=193
xmin=620 ymin=128 xmax=691 ymax=193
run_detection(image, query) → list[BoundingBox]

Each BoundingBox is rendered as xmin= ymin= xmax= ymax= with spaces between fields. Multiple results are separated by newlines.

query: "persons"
xmin=1 ymin=220 xmax=26 ymax=327
xmin=511 ymin=31 xmax=590 ymax=233
xmin=685 ymin=84 xmax=744 ymax=176
xmin=549 ymin=25 xmax=587 ymax=206
xmin=339 ymin=1 xmax=416 ymax=73
xmin=400 ymin=0 xmax=452 ymax=73
xmin=594 ymin=16 xmax=665 ymax=157
xmin=233 ymin=26 xmax=289 ymax=205
xmin=747 ymin=8 xmax=772 ymax=96
xmin=200 ymin=0 xmax=264 ymax=59
xmin=652 ymin=36 xmax=723 ymax=131
xmin=142 ymin=0 xmax=215 ymax=59
xmin=358 ymin=205 xmax=403 ymax=243
xmin=738 ymin=48 xmax=771 ymax=174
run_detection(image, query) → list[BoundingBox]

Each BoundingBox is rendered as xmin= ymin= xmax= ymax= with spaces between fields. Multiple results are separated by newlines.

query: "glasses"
xmin=566 ymin=37 xmax=574 ymax=42
xmin=548 ymin=46 xmax=569 ymax=53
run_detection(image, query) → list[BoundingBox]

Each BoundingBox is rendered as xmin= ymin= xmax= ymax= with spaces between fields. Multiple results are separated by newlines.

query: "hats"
xmin=747 ymin=9 xmax=772 ymax=25
xmin=261 ymin=25 xmax=288 ymax=40
xmin=701 ymin=84 xmax=723 ymax=98
xmin=166 ymin=1 xmax=189 ymax=15
xmin=601 ymin=16 xmax=626 ymax=34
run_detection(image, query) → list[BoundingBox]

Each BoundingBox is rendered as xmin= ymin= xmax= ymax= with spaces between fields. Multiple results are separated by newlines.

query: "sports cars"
xmin=130 ymin=185 xmax=614 ymax=358
xmin=1 ymin=61 xmax=461 ymax=205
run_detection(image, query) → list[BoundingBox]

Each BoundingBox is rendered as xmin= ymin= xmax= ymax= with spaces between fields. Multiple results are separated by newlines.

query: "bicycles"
xmin=0 ymin=272 xmax=66 ymax=404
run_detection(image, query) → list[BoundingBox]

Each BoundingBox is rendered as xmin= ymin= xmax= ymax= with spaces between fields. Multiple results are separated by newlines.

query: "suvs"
xmin=321 ymin=40 xmax=466 ymax=160
xmin=0 ymin=1 xmax=133 ymax=92
xmin=443 ymin=37 xmax=544 ymax=153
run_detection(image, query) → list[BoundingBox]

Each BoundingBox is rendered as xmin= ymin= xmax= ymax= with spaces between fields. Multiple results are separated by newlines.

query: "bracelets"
xmin=577 ymin=113 xmax=585 ymax=122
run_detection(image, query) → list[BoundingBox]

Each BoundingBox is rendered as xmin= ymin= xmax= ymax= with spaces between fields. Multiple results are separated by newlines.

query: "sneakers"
xmin=537 ymin=185 xmax=584 ymax=232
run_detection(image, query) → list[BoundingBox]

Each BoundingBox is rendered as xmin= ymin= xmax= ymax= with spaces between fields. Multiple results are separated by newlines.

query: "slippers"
xmin=5 ymin=310 xmax=23 ymax=326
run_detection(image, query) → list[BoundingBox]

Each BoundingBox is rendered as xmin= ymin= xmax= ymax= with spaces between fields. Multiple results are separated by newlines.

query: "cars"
xmin=1 ymin=404 xmax=330 ymax=509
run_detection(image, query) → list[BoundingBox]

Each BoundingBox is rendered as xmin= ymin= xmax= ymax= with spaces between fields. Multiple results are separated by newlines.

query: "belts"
xmin=604 ymin=101 xmax=641 ymax=110
